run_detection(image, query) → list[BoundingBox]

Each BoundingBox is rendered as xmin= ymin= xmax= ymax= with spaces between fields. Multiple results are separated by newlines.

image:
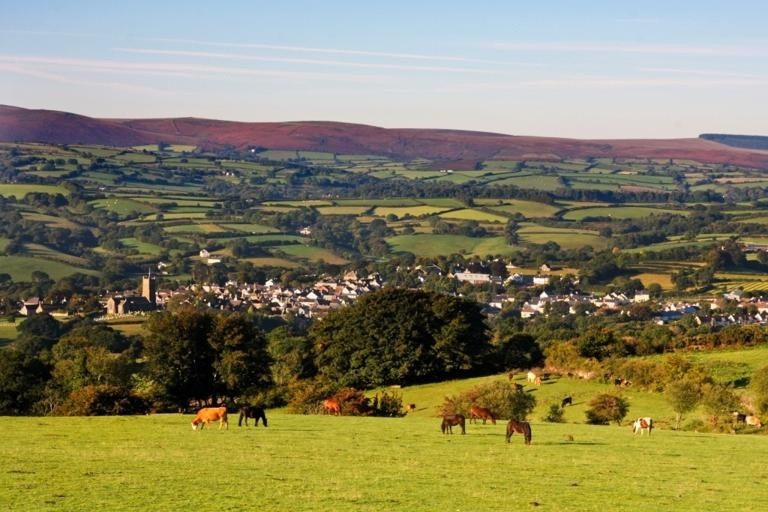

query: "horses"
xmin=632 ymin=418 xmax=652 ymax=436
xmin=191 ymin=407 xmax=228 ymax=430
xmin=406 ymin=403 xmax=416 ymax=413
xmin=238 ymin=405 xmax=267 ymax=427
xmin=440 ymin=414 xmax=465 ymax=435
xmin=528 ymin=371 xmax=551 ymax=385
xmin=470 ymin=404 xmax=496 ymax=425
xmin=506 ymin=420 xmax=531 ymax=445
xmin=323 ymin=399 xmax=342 ymax=416
xmin=508 ymin=373 xmax=513 ymax=381
xmin=720 ymin=409 xmax=763 ymax=429
xmin=615 ymin=379 xmax=622 ymax=386
xmin=561 ymin=397 xmax=572 ymax=408
